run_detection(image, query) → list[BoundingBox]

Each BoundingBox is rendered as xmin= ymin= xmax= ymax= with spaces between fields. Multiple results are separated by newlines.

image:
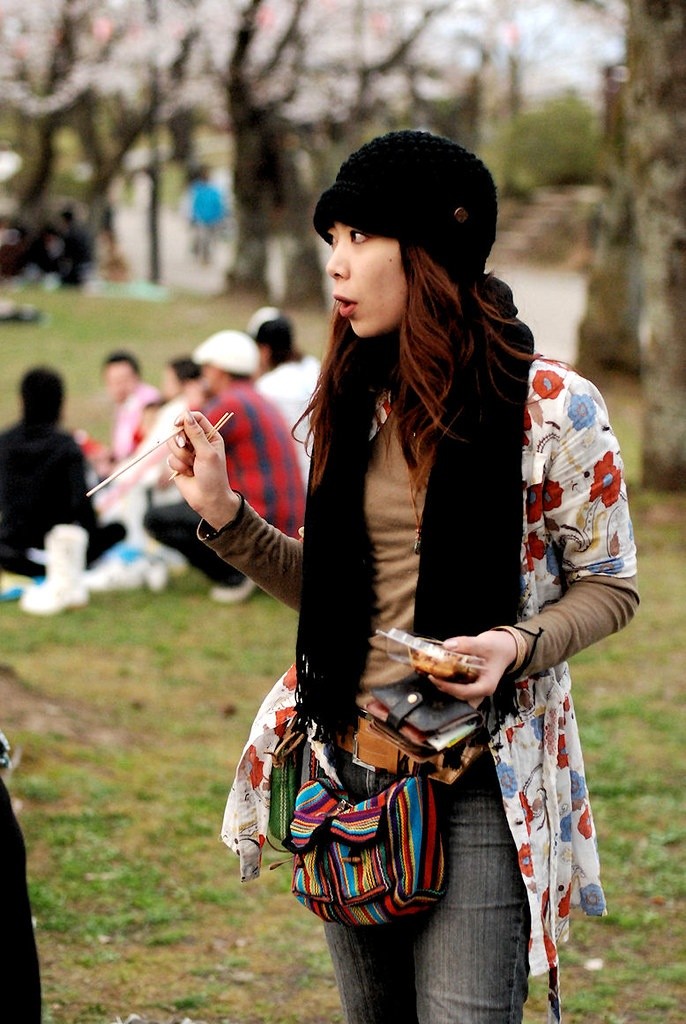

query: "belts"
xmin=325 ymin=715 xmax=486 ymax=783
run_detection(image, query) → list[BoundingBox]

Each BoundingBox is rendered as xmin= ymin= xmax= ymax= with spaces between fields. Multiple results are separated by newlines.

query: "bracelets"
xmin=505 ymin=625 xmax=543 ymax=682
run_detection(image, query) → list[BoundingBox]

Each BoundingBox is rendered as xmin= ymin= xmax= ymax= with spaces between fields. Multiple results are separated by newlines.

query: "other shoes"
xmin=211 ymin=575 xmax=256 ymax=605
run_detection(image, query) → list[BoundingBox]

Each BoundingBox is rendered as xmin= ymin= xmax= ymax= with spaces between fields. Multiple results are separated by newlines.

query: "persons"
xmin=0 ymin=209 xmax=119 ymax=280
xmin=190 ymin=163 xmax=230 ymax=261
xmin=0 ymin=369 xmax=128 ymax=581
xmin=247 ymin=306 xmax=321 ymax=488
xmin=72 ymin=352 xmax=158 ymax=484
xmin=145 ymin=331 xmax=306 ymax=605
xmin=170 ymin=130 xmax=640 ymax=1023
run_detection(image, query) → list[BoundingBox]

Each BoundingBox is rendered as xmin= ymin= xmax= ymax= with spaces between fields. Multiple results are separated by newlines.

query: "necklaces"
xmin=409 ymin=479 xmax=422 ymax=554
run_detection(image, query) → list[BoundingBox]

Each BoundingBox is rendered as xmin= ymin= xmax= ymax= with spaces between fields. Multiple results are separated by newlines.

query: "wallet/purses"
xmin=360 ymin=676 xmax=484 ymax=763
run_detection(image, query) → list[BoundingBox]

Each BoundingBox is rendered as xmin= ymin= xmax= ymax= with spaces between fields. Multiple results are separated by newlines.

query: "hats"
xmin=313 ymin=130 xmax=500 ymax=281
xmin=194 ymin=329 xmax=260 ymax=375
xmin=247 ymin=306 xmax=293 ymax=345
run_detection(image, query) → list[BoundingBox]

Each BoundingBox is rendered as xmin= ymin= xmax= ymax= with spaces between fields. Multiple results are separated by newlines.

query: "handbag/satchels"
xmin=284 ymin=775 xmax=446 ymax=924
